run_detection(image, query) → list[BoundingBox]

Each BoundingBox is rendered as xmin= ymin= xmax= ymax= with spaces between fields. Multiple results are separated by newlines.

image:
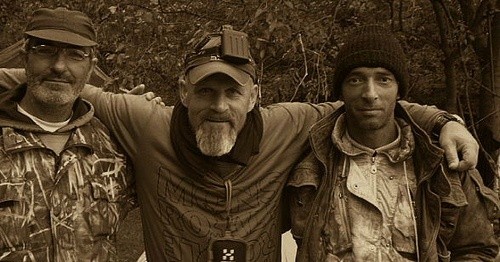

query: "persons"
xmin=0 ymin=6 xmax=165 ymax=262
xmin=281 ymin=25 xmax=500 ymax=262
xmin=1 ymin=29 xmax=480 ymax=262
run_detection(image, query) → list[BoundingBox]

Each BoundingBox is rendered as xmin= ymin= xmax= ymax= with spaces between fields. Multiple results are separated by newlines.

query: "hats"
xmin=330 ymin=23 xmax=409 ymax=102
xmin=24 ymin=7 xmax=99 ymax=48
xmin=186 ymin=24 xmax=257 ymax=87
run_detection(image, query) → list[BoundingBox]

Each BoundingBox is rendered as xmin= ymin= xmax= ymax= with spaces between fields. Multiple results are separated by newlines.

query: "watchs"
xmin=431 ymin=113 xmax=466 ymax=145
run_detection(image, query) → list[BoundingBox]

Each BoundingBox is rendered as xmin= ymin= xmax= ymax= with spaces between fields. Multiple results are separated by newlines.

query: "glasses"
xmin=26 ymin=43 xmax=95 ymax=63
